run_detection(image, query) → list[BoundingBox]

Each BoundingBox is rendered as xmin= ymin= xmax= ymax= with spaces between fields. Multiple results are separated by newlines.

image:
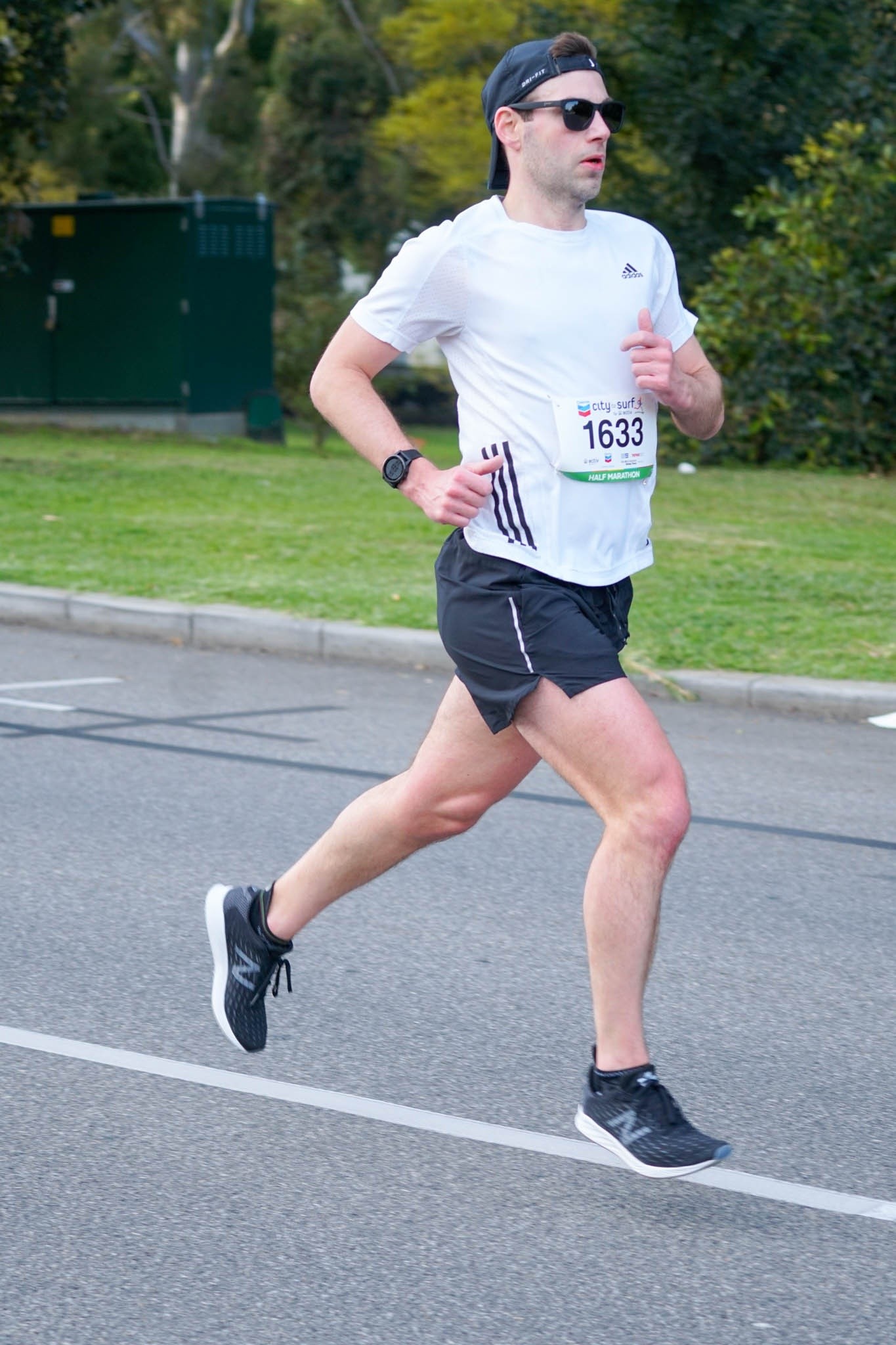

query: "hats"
xmin=480 ymin=37 xmax=605 ymax=193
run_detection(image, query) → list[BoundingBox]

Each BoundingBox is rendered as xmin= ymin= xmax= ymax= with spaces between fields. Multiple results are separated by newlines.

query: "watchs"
xmin=382 ymin=448 xmax=422 ymax=488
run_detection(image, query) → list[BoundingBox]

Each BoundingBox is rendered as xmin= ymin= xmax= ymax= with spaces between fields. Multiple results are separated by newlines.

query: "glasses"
xmin=492 ymin=99 xmax=627 ymax=133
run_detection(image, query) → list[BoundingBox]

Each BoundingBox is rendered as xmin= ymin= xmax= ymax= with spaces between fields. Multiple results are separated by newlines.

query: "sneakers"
xmin=571 ymin=1044 xmax=732 ymax=1177
xmin=205 ymin=877 xmax=292 ymax=1055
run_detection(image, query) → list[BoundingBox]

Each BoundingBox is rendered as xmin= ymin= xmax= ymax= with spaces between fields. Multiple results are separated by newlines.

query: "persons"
xmin=203 ymin=33 xmax=735 ymax=1178
